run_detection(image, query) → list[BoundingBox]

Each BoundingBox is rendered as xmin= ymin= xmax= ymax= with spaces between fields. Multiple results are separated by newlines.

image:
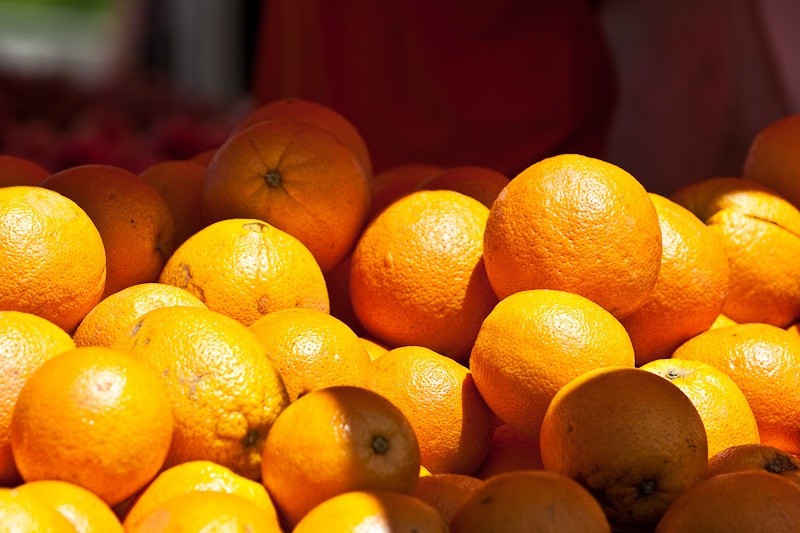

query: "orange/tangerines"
xmin=0 ymin=96 xmax=800 ymax=533
